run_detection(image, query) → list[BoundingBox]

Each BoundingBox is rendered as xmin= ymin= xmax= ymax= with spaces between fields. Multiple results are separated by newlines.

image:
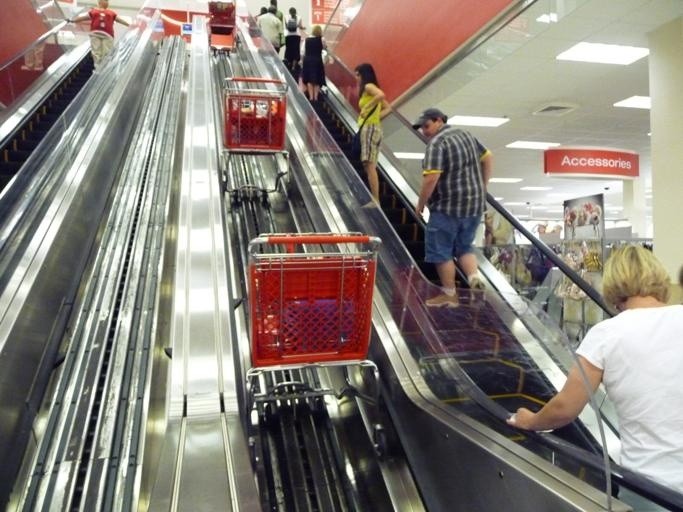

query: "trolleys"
xmin=208 ymin=2 xmax=236 ymax=59
xmin=242 ymin=230 xmax=390 ymax=473
xmin=218 ymin=74 xmax=294 ymax=208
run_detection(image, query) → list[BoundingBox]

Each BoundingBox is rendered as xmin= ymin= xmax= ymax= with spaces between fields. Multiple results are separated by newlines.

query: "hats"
xmin=411 ymin=107 xmax=448 ymax=129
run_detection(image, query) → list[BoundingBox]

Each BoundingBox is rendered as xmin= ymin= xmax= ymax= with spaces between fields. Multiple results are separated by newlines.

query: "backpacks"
xmin=286 ymin=18 xmax=296 ymax=32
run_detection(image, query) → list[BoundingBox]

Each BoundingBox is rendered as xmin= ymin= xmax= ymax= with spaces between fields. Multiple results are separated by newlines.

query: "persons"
xmin=508 ymin=243 xmax=683 ymax=512
xmin=413 ymin=108 xmax=493 ymax=308
xmin=254 ymin=0 xmax=327 ymax=101
xmin=484 ymin=227 xmax=494 ymax=260
xmin=65 ymin=0 xmax=130 ymax=68
xmin=21 ymin=39 xmax=45 ymax=71
xmin=355 ymin=64 xmax=394 ymax=209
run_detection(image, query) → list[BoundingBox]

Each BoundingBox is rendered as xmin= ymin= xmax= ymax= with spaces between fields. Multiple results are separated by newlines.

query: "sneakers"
xmin=425 ymin=294 xmax=460 ymax=308
xmin=469 ymin=278 xmax=486 ymax=309
xmin=361 ymin=201 xmax=381 ymax=210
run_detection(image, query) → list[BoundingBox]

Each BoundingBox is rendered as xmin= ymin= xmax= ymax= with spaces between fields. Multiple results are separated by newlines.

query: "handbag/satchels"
xmin=351 ymin=133 xmax=362 ymax=160
